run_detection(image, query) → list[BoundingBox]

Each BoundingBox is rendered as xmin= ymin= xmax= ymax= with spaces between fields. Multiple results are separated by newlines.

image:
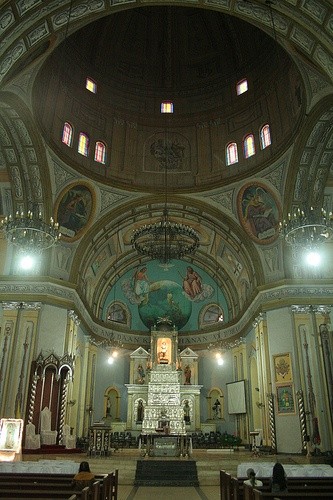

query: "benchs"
xmin=0 ymin=468 xmax=119 ymax=500
xmin=220 ymin=470 xmax=333 ymax=500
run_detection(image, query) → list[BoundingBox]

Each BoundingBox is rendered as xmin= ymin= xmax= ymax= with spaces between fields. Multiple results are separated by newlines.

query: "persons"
xmin=71 ymin=461 xmax=95 ymax=492
xmin=243 ymin=469 xmax=263 ymax=487
xmin=269 ymin=463 xmax=289 ymax=500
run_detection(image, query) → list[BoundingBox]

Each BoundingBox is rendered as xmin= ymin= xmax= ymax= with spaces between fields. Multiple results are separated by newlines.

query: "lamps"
xmin=100 ymin=224 xmax=124 ymax=352
xmin=205 ymin=224 xmax=231 ymax=355
xmin=132 ymin=103 xmax=200 ymax=263
xmin=278 ymin=206 xmax=333 ymax=248
xmin=0 ymin=210 xmax=62 ymax=254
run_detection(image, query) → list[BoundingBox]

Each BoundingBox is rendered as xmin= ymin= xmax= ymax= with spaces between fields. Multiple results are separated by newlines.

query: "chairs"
xmin=40 ymin=406 xmax=58 ymax=445
xmin=192 ymin=431 xmax=242 ymax=448
xmin=62 ymin=424 xmax=76 ymax=449
xmin=76 ymin=431 xmax=136 ymax=448
xmin=24 ymin=423 xmax=41 ymax=449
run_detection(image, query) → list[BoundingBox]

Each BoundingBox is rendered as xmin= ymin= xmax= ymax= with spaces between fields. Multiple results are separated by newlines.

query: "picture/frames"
xmin=276 ymin=384 xmax=296 ymax=416
xmin=273 ymin=352 xmax=293 ymax=384
